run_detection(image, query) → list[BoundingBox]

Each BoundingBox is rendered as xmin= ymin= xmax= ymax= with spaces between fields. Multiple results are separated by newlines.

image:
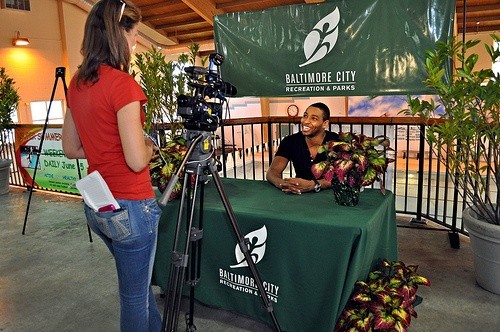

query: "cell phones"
xmin=99 ymin=204 xmax=115 ymax=211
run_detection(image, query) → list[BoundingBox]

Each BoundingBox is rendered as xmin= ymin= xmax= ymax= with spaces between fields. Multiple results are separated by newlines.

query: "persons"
xmin=62 ymin=0 xmax=163 ymax=332
xmin=265 ymin=103 xmax=342 ymax=195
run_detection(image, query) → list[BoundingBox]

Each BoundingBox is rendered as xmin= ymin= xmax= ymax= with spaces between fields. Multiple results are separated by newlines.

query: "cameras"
xmin=55 ymin=67 xmax=65 ymax=77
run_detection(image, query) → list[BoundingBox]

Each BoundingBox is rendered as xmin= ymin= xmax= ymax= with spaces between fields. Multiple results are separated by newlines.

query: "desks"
xmin=152 ymin=176 xmax=398 ymax=332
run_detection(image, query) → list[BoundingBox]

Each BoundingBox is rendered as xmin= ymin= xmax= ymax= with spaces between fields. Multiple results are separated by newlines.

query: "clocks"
xmin=287 ymin=105 xmax=299 ymax=117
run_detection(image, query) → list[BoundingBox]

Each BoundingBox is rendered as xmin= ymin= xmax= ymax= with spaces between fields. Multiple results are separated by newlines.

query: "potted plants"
xmin=395 ymin=33 xmax=500 ymax=293
xmin=311 ymin=131 xmax=398 ymax=206
xmin=0 ymin=66 xmax=22 ymax=195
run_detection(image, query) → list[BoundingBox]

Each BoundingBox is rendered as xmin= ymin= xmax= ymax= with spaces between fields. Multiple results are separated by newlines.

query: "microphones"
xmin=220 ymin=82 xmax=238 ymax=96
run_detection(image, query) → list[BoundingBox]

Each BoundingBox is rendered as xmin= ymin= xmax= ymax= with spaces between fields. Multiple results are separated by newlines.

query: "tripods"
xmin=160 ymin=131 xmax=282 ymax=332
xmin=22 ymin=76 xmax=93 ymax=244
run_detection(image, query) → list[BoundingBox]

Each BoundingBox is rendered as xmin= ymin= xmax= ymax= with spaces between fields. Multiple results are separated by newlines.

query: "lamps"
xmin=12 ymin=31 xmax=30 ymax=47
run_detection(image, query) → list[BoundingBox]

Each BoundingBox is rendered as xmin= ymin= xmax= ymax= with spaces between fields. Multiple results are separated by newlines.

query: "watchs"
xmin=313 ymin=179 xmax=321 ymax=192
